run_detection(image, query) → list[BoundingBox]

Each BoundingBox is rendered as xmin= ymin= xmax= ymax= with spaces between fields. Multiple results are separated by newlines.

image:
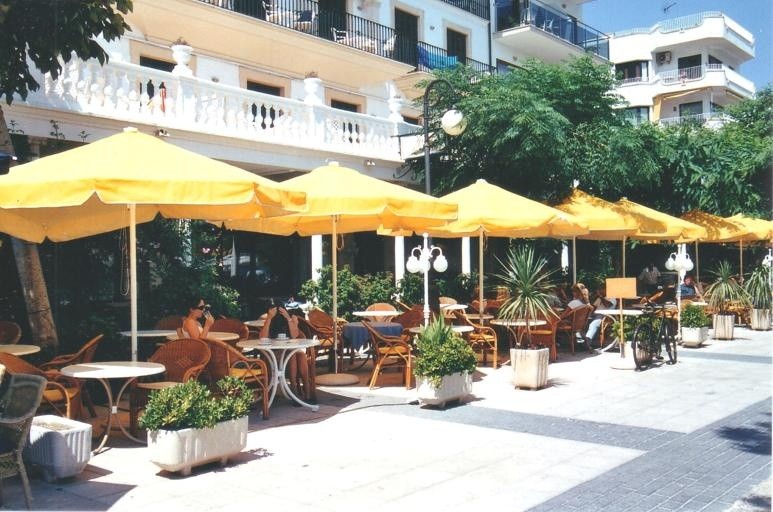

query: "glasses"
xmin=195 ymin=305 xmax=204 ymax=310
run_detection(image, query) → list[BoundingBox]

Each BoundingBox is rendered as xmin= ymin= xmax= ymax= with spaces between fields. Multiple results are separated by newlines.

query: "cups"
xmin=277 ymin=333 xmax=286 ymax=338
xmin=259 ymin=337 xmax=270 ymax=343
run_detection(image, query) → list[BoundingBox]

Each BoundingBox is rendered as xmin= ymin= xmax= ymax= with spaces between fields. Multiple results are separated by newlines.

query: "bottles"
xmin=312 ymin=334 xmax=317 ymax=344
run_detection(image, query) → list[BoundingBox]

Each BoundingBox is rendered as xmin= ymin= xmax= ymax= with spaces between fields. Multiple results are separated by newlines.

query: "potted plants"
xmin=701 ymin=257 xmax=755 ymax=339
xmin=480 ymin=244 xmax=569 ymax=393
xmin=137 ymin=375 xmax=255 ymax=476
xmin=745 ymin=262 xmax=771 ymax=331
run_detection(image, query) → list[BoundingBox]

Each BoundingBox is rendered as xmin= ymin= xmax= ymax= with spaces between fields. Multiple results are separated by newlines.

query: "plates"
xmin=257 ymin=342 xmax=272 ymax=346
xmin=275 ymin=337 xmax=289 ymax=341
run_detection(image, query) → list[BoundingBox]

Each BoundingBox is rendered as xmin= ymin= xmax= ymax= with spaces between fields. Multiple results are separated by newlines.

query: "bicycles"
xmin=631 ymin=285 xmax=677 ymax=371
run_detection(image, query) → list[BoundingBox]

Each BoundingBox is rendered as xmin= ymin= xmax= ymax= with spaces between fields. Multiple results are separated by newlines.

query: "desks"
xmin=57 ymin=360 xmax=166 ymax=454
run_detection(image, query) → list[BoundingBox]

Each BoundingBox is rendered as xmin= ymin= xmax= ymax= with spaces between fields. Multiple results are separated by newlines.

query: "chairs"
xmin=0 ymin=372 xmax=48 ymax=511
xmin=202 ymin=0 xmax=399 ymax=59
xmin=517 ymin=3 xmax=559 ymax=37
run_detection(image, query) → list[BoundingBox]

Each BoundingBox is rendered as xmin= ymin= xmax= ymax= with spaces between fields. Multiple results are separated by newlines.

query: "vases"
xmin=23 ymin=413 xmax=93 ymax=482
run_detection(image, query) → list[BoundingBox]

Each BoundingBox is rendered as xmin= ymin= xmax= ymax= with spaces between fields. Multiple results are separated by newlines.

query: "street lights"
xmin=421 ymin=78 xmax=467 ymax=320
xmin=761 ymin=247 xmax=773 ymax=289
xmin=666 ymin=242 xmax=696 ymax=300
xmin=406 ymin=230 xmax=448 ymax=330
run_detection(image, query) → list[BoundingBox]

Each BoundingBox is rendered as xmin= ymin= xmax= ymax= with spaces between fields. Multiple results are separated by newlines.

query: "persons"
xmin=678 ymin=65 xmax=689 ymax=84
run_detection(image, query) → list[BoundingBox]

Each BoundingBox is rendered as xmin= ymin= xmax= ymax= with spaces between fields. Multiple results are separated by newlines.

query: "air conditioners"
xmin=655 ymin=51 xmax=670 ymax=65
